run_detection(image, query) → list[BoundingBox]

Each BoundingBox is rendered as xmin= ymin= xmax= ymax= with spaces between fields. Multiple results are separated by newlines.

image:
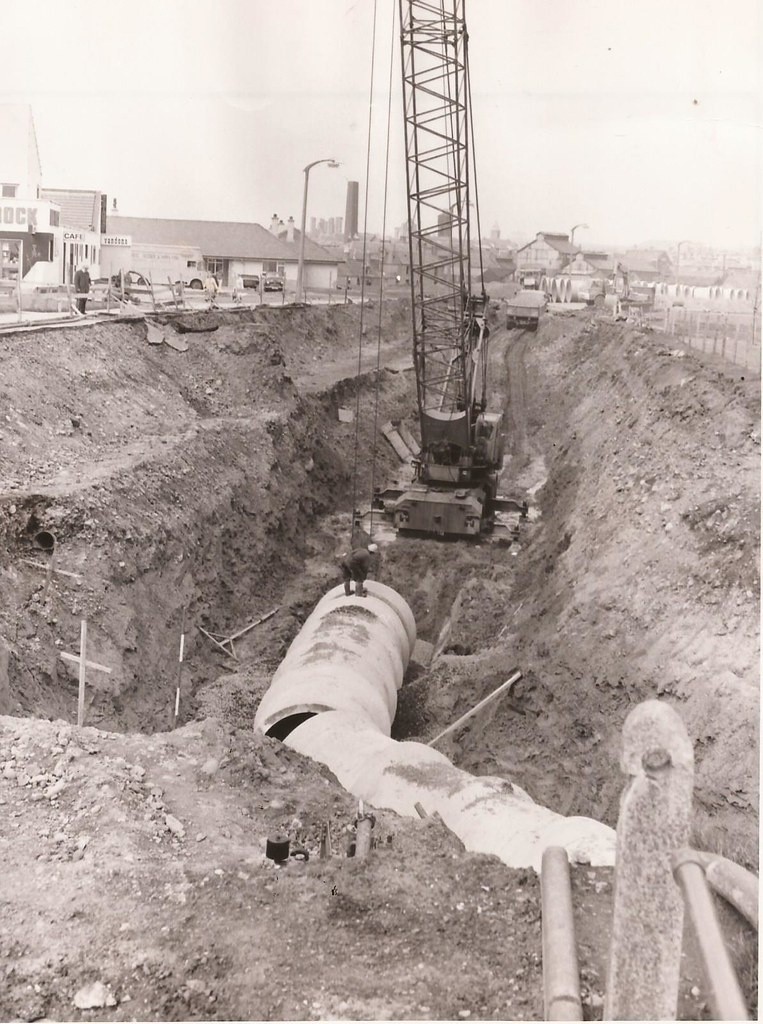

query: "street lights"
xmin=294 ymin=158 xmax=342 ymax=303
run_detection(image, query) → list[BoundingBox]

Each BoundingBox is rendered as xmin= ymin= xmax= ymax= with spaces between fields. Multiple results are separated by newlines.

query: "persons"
xmin=339 ymin=543 xmax=378 ymax=596
xmin=202 ymin=267 xmax=245 ymax=308
xmin=74 ymin=264 xmax=93 ymax=316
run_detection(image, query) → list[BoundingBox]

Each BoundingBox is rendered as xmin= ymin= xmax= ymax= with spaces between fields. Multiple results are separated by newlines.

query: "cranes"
xmin=340 ymin=0 xmax=490 ymax=599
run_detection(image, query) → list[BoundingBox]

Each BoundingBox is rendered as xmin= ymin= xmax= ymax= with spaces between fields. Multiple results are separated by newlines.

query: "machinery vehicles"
xmin=614 ymin=262 xmax=652 ymax=315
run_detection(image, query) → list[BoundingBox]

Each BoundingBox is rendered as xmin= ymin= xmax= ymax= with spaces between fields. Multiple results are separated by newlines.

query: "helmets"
xmin=368 ymin=543 xmax=378 ymax=553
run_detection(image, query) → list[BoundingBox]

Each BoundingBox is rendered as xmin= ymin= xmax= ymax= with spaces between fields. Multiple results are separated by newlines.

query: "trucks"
xmin=506 ymin=289 xmax=552 ymax=331
xmin=131 ymin=242 xmax=208 ymax=290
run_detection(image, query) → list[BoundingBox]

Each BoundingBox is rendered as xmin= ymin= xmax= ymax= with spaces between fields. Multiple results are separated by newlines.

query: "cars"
xmin=264 ymin=277 xmax=283 ymax=291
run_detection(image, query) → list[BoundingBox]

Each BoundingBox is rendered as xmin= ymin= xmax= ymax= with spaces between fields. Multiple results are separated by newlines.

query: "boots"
xmin=355 ymin=581 xmax=366 ymax=597
xmin=345 ymin=580 xmax=354 ymax=596
